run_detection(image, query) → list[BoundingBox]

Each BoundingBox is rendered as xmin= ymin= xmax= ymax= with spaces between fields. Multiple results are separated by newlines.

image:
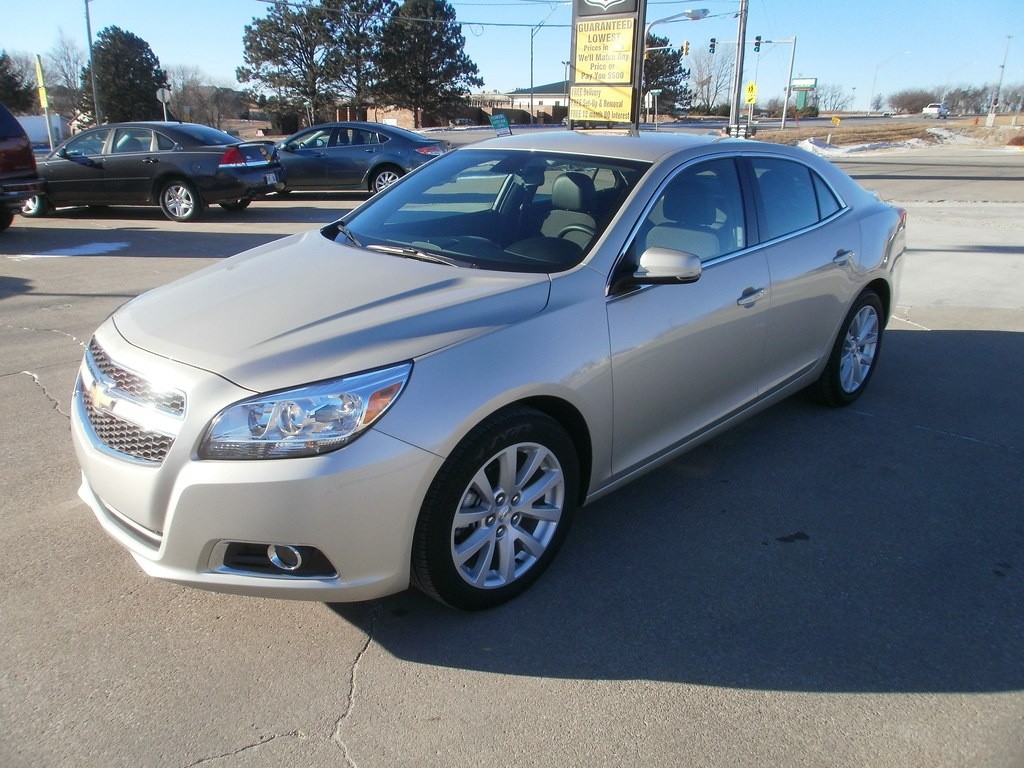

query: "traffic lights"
xmin=754 ymin=36 xmax=761 ymax=52
xmin=683 ymin=41 xmax=689 ymax=54
xmin=709 ymin=38 xmax=716 ymax=54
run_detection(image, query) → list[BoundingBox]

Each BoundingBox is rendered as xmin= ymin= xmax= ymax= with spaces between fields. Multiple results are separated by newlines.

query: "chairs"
xmin=539 ymin=172 xmax=600 ymax=247
xmin=644 ymin=179 xmax=724 ymax=265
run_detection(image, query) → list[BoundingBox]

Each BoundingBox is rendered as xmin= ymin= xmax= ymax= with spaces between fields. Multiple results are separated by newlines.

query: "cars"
xmin=71 ymin=134 xmax=908 ymax=611
xmin=20 ymin=121 xmax=287 ymax=222
xmin=562 ymin=116 xmax=614 ymax=129
xmin=252 ymin=123 xmax=457 ymax=200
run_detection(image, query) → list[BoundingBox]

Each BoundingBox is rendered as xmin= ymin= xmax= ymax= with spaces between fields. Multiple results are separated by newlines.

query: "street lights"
xmin=562 ymin=60 xmax=570 ymax=110
xmin=639 ymin=8 xmax=710 ymax=113
xmin=866 ymin=52 xmax=911 ymax=117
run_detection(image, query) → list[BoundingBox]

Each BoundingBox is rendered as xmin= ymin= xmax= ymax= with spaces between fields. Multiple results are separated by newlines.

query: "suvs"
xmin=0 ymin=104 xmax=48 ymax=231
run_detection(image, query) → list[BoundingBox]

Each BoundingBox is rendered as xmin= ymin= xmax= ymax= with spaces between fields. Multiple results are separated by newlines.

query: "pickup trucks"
xmin=923 ymin=104 xmax=948 ymax=119
